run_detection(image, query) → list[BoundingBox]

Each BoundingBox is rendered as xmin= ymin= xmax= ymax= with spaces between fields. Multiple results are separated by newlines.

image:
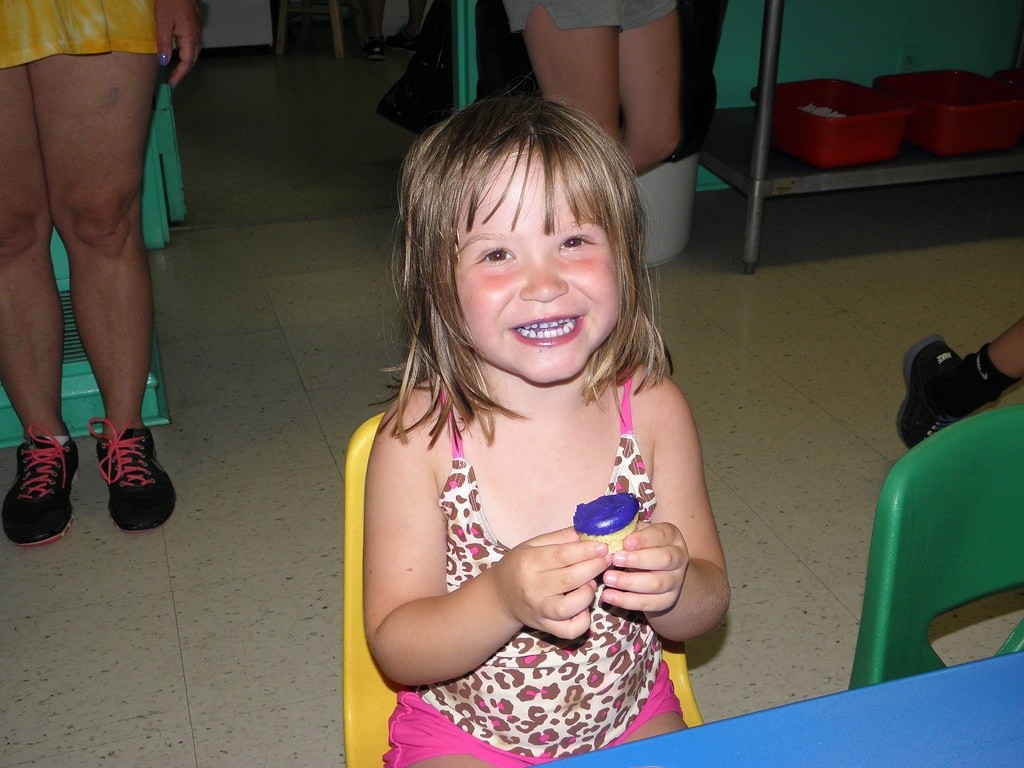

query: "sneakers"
xmin=361 ymin=39 xmax=386 ymax=59
xmin=88 ymin=417 xmax=177 ymax=532
xmin=386 ymin=23 xmax=420 ymax=54
xmin=2 ymin=421 xmax=79 ymax=547
xmin=896 ymin=335 xmax=963 ymax=450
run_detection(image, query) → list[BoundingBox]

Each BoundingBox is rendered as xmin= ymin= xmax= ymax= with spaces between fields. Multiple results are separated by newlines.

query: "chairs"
xmin=848 ymin=405 xmax=1024 ymax=691
xmin=342 ymin=413 xmax=703 ymax=768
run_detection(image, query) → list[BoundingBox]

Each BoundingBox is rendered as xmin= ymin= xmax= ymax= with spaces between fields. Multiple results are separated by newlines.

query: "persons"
xmin=504 ymin=0 xmax=681 ymax=178
xmin=360 ymin=0 xmax=428 ymax=59
xmin=364 ymin=95 xmax=731 ymax=768
xmin=895 ymin=317 xmax=1024 ymax=448
xmin=0 ymin=0 xmax=204 ymax=546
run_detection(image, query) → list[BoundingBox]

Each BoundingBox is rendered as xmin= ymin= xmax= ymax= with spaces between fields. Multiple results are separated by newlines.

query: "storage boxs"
xmin=750 ymin=77 xmax=920 ymax=166
xmin=874 ymin=68 xmax=1024 ymax=159
